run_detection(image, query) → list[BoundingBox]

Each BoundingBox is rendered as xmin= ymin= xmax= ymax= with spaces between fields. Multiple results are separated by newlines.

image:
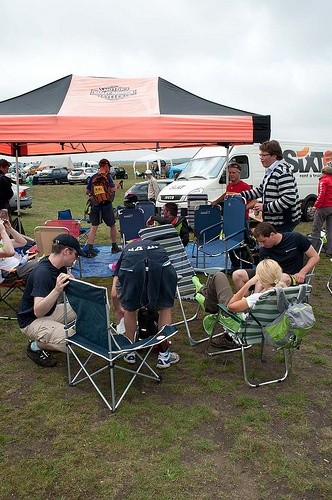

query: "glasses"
xmin=259 ymin=154 xmax=270 ymax=158
xmin=228 ymin=165 xmax=238 ymax=167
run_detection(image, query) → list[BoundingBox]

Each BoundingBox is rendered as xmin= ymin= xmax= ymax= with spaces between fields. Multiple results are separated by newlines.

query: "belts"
xmin=128 ymin=245 xmax=158 ymax=251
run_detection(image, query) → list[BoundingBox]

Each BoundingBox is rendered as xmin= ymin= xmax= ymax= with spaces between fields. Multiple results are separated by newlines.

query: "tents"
xmin=0 ymin=74 xmax=271 ymax=234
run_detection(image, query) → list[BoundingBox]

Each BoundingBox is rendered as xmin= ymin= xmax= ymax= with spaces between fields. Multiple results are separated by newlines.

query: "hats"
xmin=99 ymin=158 xmax=113 ymax=167
xmin=145 ymin=170 xmax=152 ymax=174
xmin=53 ymin=234 xmax=84 ymax=256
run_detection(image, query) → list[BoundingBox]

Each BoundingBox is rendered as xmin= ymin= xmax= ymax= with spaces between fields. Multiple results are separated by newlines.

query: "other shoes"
xmin=227 ymin=270 xmax=234 ymax=277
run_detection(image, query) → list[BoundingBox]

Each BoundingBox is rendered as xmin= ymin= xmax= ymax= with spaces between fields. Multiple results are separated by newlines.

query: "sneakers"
xmin=124 ymin=352 xmax=136 ymax=363
xmin=112 ymin=247 xmax=121 ymax=254
xmin=211 ymin=334 xmax=236 ymax=348
xmin=27 ymin=340 xmax=58 ymax=367
xmin=156 ymin=352 xmax=179 ymax=368
xmin=88 ymin=252 xmax=97 ymax=258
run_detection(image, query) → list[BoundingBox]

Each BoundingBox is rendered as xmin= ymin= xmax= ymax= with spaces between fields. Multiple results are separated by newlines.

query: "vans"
xmin=155 ymin=139 xmax=332 ymax=227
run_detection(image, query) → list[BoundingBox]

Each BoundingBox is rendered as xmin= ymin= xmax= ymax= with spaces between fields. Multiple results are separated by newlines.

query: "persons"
xmin=0 ymin=220 xmax=40 ymax=280
xmin=17 ymin=234 xmax=80 ymax=367
xmin=232 ymin=222 xmax=320 ymax=297
xmin=190 ymin=259 xmax=288 ymax=333
xmin=231 ymin=142 xmax=303 ymax=233
xmin=311 ymin=166 xmax=332 ymax=257
xmin=211 ymin=163 xmax=256 ymax=230
xmin=0 ymin=157 xmax=188 ymax=254
xmin=110 ymin=239 xmax=179 ymax=369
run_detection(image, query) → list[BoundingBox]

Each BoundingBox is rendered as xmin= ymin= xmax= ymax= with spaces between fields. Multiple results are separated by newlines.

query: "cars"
xmin=9 ymin=183 xmax=32 ymax=212
xmin=124 ymin=179 xmax=172 ymax=207
xmin=109 ymin=167 xmax=128 ymax=180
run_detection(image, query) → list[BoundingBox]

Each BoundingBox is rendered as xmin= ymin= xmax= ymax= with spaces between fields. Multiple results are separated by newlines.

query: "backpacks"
xmin=262 ymin=284 xmax=316 ymax=349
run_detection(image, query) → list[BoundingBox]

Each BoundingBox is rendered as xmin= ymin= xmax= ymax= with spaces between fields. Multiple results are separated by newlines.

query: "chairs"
xmin=197 ymin=191 xmax=249 ymax=273
xmin=290 ymin=234 xmax=324 ymax=297
xmin=0 ymin=266 xmax=25 ymax=320
xmin=231 ymin=281 xmax=316 ymax=387
xmin=119 ymin=208 xmax=144 ymax=250
xmin=61 ymin=270 xmax=178 ymax=412
xmin=144 ymin=223 xmax=226 ymax=345
xmin=44 ymin=218 xmax=81 ymax=245
xmin=32 ymin=224 xmax=85 ymax=278
xmin=191 ymin=203 xmax=222 ymax=258
xmin=135 ymin=200 xmax=157 ymax=225
xmin=57 ymin=209 xmax=91 ymax=240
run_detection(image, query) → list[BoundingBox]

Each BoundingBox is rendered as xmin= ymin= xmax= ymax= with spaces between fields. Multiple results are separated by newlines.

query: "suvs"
xmin=66 ymin=167 xmax=98 ymax=184
xmin=39 ymin=167 xmax=71 ymax=185
